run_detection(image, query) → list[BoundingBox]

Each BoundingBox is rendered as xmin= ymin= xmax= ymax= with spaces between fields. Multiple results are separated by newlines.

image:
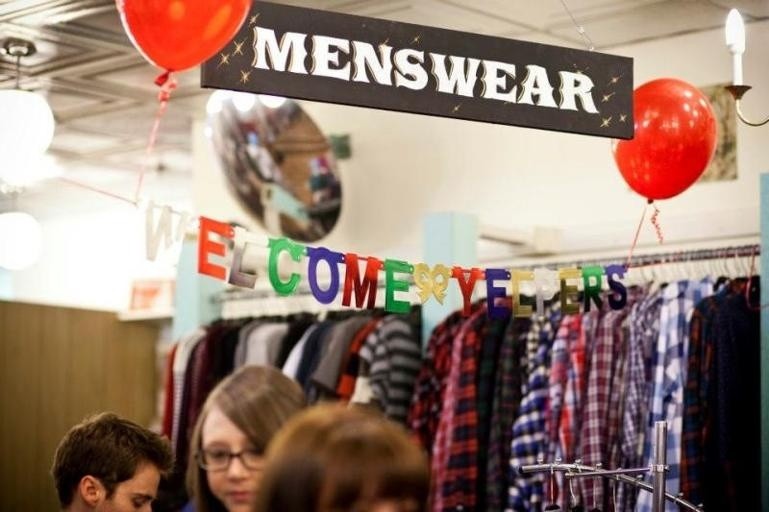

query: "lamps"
xmin=0 ymin=191 xmax=42 ymax=270
xmin=724 ymin=8 xmax=769 ymax=127
xmin=0 ymin=37 xmax=55 ymax=171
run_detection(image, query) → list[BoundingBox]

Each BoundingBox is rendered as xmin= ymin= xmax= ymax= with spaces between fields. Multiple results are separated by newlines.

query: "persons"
xmin=254 ymin=400 xmax=432 ymax=512
xmin=50 ymin=412 xmax=177 ymax=512
xmin=178 ymin=365 xmax=308 ymax=512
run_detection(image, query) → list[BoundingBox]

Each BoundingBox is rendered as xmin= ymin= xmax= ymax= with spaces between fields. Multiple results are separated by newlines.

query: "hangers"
xmin=502 ymin=245 xmax=760 ymax=310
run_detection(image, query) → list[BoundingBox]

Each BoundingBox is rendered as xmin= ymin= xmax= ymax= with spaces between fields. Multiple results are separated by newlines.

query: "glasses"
xmin=192 ymin=444 xmax=270 ymax=472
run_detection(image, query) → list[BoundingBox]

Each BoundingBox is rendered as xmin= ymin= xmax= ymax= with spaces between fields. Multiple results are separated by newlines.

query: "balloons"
xmin=114 ymin=0 xmax=256 ymax=86
xmin=612 ymin=76 xmax=719 ymax=205
